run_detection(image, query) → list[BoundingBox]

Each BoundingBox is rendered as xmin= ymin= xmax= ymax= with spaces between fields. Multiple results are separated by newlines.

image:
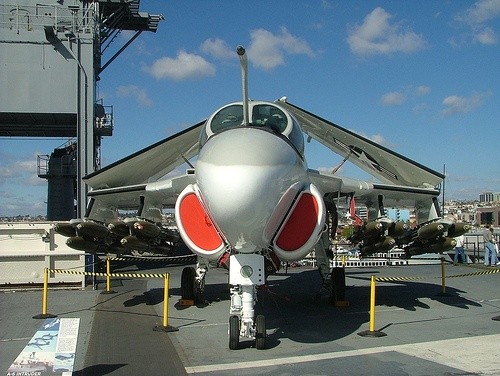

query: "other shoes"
xmin=483 ymin=262 xmax=489 ymax=266
xmin=491 ymin=265 xmax=494 ymax=267
xmin=496 ymin=262 xmax=500 ymax=265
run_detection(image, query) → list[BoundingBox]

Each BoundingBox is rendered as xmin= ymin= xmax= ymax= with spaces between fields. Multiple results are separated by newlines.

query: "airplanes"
xmin=52 ymin=43 xmax=472 ymax=351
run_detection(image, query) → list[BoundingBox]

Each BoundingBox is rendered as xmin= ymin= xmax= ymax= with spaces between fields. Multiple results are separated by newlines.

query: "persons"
xmin=483 ymin=224 xmax=500 ymax=267
xmin=453 ymin=235 xmax=467 ymax=264
